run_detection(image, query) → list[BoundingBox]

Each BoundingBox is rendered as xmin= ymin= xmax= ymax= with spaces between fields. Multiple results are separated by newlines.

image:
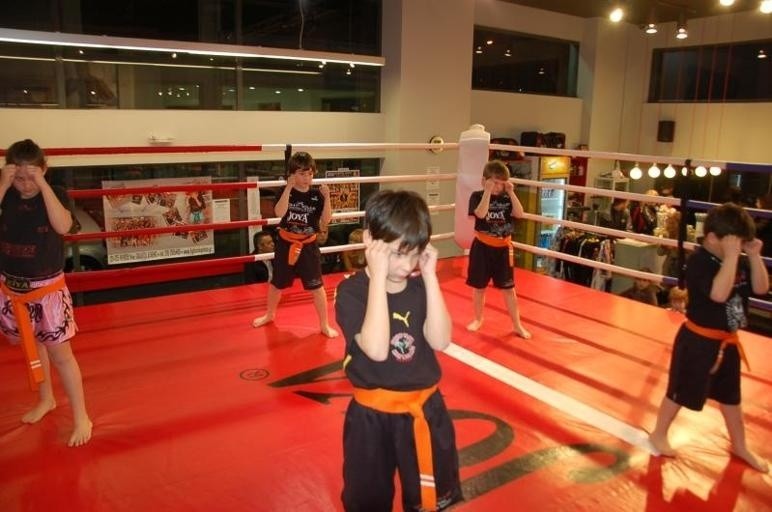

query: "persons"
xmin=1 ymin=138 xmax=94 ymax=447
xmin=316 ymin=227 xmax=342 ymax=271
xmin=253 ymin=153 xmax=339 ymax=338
xmin=185 ymin=190 xmax=207 ymax=238
xmin=332 ymin=189 xmax=468 ymax=511
xmin=551 ymin=189 xmax=771 ymax=339
xmin=338 ymin=229 xmax=371 ymax=271
xmin=241 ymin=232 xmax=276 ymax=284
xmin=648 ymin=201 xmax=769 ymax=473
xmin=465 ymin=160 xmax=532 ymax=339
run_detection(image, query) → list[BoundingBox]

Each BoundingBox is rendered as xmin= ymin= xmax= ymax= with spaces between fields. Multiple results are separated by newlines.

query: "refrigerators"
xmin=507 ymin=156 xmax=570 ymax=275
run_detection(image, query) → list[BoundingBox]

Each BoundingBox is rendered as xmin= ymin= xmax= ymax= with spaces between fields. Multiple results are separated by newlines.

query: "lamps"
xmin=674 ymin=10 xmax=690 ymax=40
